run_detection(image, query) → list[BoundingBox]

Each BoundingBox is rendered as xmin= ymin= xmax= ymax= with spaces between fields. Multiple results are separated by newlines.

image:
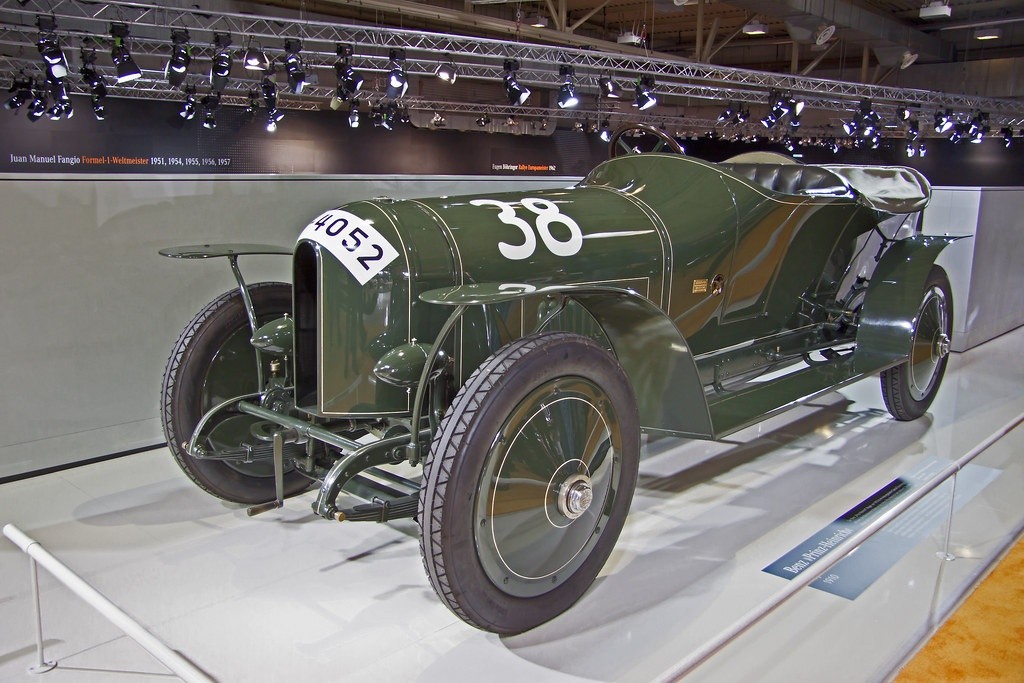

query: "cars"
xmin=157 ymin=122 xmax=974 ymax=636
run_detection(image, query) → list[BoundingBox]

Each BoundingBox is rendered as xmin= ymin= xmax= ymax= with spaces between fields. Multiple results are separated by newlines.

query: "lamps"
xmin=717 ymin=101 xmax=752 ymax=125
xmin=435 ymin=52 xmax=462 ymax=84
xmin=432 ymin=112 xmax=446 ymax=127
xmin=677 ymin=129 xmax=687 ymax=140
xmin=590 ymin=121 xmax=598 ymax=133
xmin=572 ymin=120 xmax=584 ymax=132
xmin=633 ymin=124 xmax=667 ymax=137
xmin=502 ymin=114 xmax=549 ymax=132
xmin=501 ymin=61 xmax=532 ymax=106
xmin=627 ymin=130 xmax=631 ymax=136
xmin=475 ymin=114 xmax=492 ymax=127
xmin=632 ymin=75 xmax=657 ymax=110
xmin=705 ymin=128 xmax=719 ymax=138
xmin=3 ymin=14 xmax=411 ymax=132
xmin=599 ymin=70 xmax=623 ymax=99
xmin=557 ymin=66 xmax=580 ymax=108
xmin=760 ymin=94 xmax=1024 ymax=158
xmin=689 ymin=130 xmax=699 ymax=140
xmin=599 ymin=119 xmax=612 ymax=143
xmin=721 ymin=130 xmax=758 ymax=143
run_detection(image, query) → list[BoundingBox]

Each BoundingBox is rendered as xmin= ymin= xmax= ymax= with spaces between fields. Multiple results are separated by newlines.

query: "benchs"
xmin=713 ymin=161 xmax=847 ymax=197
